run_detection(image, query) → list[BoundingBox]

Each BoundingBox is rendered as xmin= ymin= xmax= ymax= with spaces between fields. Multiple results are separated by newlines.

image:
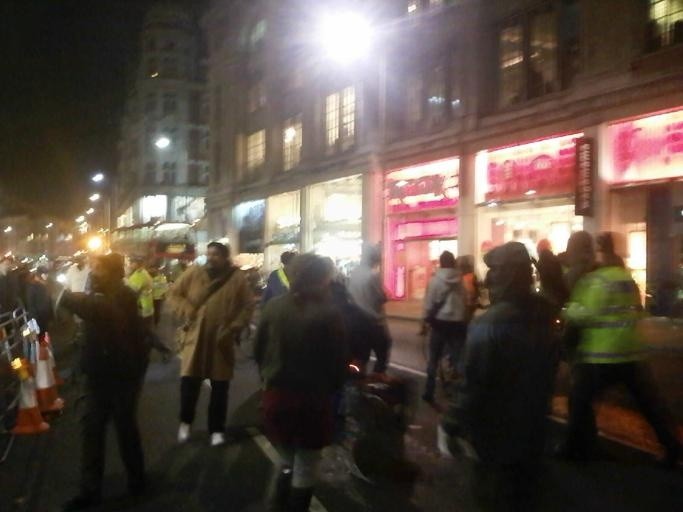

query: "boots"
xmin=268 ymin=466 xmax=313 ymax=512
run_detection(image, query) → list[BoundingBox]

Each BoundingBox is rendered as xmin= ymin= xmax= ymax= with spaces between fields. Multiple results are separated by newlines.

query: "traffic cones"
xmin=7 ymin=329 xmax=66 ymax=438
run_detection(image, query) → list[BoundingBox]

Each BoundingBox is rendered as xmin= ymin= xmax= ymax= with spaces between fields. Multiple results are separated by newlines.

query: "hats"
xmin=484 ymin=242 xmax=530 ymax=267
xmin=99 ymin=253 xmax=125 ymax=277
xmin=441 ymin=250 xmax=456 ymax=270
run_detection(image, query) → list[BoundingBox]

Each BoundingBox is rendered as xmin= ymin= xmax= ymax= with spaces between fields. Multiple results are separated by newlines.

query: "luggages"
xmin=346 ymin=374 xmax=415 ymax=484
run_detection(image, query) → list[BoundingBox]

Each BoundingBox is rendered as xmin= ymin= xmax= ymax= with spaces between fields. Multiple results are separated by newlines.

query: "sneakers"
xmin=437 ymin=422 xmax=458 ymax=459
xmin=177 ymin=421 xmax=189 ymax=442
xmin=422 ymin=392 xmax=433 ymax=401
xmin=210 ymin=431 xmax=225 ymax=446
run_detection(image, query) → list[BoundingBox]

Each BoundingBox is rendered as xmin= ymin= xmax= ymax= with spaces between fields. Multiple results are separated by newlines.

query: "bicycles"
xmin=236 ymin=319 xmax=260 ymax=361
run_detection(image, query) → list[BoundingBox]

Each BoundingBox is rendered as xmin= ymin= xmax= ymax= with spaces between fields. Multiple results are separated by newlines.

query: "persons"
xmin=2 ymin=233 xmax=413 ymax=512
xmin=418 ymin=233 xmax=682 ymax=511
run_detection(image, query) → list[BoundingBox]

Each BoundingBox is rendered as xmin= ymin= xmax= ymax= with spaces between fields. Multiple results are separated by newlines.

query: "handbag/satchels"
xmin=174 ymin=323 xmax=186 ymax=353
xmin=426 ymin=305 xmax=440 ymax=324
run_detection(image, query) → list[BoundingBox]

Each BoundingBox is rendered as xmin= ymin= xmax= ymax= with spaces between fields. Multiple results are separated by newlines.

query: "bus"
xmin=108 ymin=219 xmax=195 ymax=283
